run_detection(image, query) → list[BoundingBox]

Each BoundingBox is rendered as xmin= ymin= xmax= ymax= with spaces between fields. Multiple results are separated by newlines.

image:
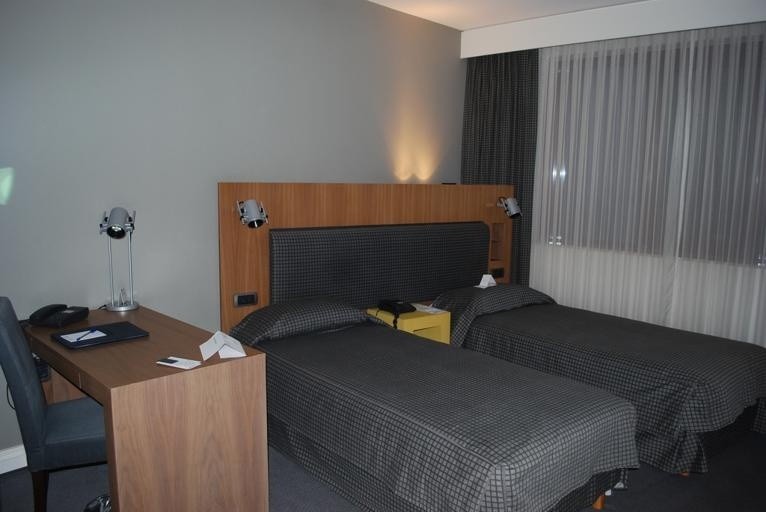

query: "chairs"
xmin=0 ymin=294 xmax=107 ymax=511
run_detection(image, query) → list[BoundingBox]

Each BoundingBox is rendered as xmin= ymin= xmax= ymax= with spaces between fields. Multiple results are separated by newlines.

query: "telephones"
xmin=19 ymin=304 xmax=88 ymax=328
xmin=378 ymin=300 xmax=416 ymax=315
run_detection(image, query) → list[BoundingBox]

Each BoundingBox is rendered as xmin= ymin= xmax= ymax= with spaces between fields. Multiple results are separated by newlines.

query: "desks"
xmin=23 ymin=301 xmax=271 ymax=512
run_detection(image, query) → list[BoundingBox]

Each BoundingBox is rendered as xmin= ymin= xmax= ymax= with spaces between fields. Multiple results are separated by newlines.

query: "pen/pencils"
xmin=77 ymin=329 xmax=96 ymax=340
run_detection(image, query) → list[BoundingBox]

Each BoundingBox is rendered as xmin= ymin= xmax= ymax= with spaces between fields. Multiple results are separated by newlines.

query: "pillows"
xmin=432 ymin=282 xmax=558 ymax=347
xmin=228 ymin=296 xmax=391 ymax=347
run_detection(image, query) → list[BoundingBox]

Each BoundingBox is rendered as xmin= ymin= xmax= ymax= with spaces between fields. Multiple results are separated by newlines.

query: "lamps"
xmin=497 ymin=196 xmax=524 ymax=220
xmin=98 ymin=206 xmax=140 ymax=312
xmin=237 ymin=198 xmax=269 ymax=229
